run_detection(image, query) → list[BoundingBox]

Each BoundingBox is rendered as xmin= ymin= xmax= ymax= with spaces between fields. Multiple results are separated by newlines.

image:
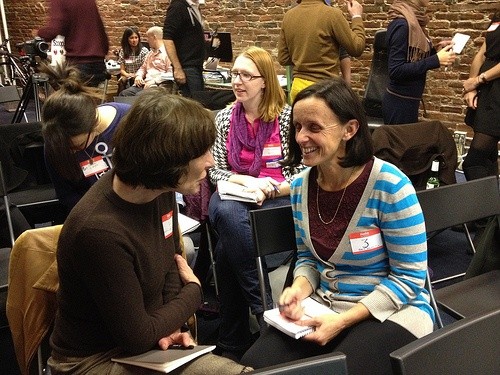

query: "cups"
xmin=426 ymin=160 xmax=440 ymax=190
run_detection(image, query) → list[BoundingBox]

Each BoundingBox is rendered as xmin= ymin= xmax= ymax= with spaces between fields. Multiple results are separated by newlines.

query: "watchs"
xmin=480 ymin=73 xmax=489 ymax=82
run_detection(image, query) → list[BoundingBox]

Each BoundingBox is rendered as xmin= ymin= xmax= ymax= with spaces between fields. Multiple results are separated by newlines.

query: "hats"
xmin=203 ymin=57 xmax=221 ymax=71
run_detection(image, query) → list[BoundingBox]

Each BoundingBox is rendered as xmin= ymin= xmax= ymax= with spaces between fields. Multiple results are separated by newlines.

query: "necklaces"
xmin=83 ymin=132 xmax=100 ymax=165
xmin=316 ymin=166 xmax=355 ymax=224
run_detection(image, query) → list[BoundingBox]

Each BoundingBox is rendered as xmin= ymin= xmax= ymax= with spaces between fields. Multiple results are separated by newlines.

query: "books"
xmin=262 ymin=296 xmax=340 ymax=340
xmin=110 ymin=344 xmax=216 ymax=373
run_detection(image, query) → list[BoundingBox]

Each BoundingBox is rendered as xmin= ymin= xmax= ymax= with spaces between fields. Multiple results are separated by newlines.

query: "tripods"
xmin=11 ymin=69 xmax=48 ymax=124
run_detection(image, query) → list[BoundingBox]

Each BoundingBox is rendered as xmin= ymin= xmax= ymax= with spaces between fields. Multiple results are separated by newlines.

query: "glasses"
xmin=70 ymin=130 xmax=91 ymax=152
xmin=227 ymin=69 xmax=263 ymax=82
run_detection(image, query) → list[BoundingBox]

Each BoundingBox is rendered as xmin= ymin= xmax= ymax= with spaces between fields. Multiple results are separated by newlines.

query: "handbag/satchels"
xmin=464 ymin=106 xmax=476 ymax=130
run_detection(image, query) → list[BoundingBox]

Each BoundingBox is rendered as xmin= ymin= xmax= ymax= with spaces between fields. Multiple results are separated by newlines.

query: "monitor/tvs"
xmin=204 ymin=32 xmax=232 ymax=72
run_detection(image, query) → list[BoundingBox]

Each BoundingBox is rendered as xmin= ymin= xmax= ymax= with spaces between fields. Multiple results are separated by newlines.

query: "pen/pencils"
xmin=273 ymin=185 xmax=281 ymax=194
xmin=158 ymin=345 xmax=195 ymax=349
xmin=279 ymin=297 xmax=298 ymax=310
xmin=445 ymin=46 xmax=453 ymax=72
xmin=465 ymin=90 xmax=481 ymax=111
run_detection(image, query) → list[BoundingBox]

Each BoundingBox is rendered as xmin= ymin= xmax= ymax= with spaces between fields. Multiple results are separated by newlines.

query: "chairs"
xmin=1 ymin=86 xmax=500 ymax=375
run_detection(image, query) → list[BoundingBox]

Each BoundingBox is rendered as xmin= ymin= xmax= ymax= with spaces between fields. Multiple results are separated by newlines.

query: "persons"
xmin=239 ymin=77 xmax=436 ymax=375
xmin=162 ymin=0 xmax=206 ymax=105
xmin=382 ymin=0 xmax=458 ymax=126
xmin=32 ymin=0 xmax=112 ymax=87
xmin=38 ymin=59 xmax=134 ymax=225
xmin=117 ymin=28 xmax=150 ymax=95
xmin=451 ymin=8 xmax=500 ymax=232
xmin=119 ymin=26 xmax=187 ymax=97
xmin=205 ymin=45 xmax=310 ymax=335
xmin=278 ymin=0 xmax=366 ymax=105
xmin=44 ymin=91 xmax=256 ymax=375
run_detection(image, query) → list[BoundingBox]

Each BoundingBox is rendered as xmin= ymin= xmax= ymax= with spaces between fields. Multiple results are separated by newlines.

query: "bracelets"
xmin=478 ymin=76 xmax=482 ymax=85
